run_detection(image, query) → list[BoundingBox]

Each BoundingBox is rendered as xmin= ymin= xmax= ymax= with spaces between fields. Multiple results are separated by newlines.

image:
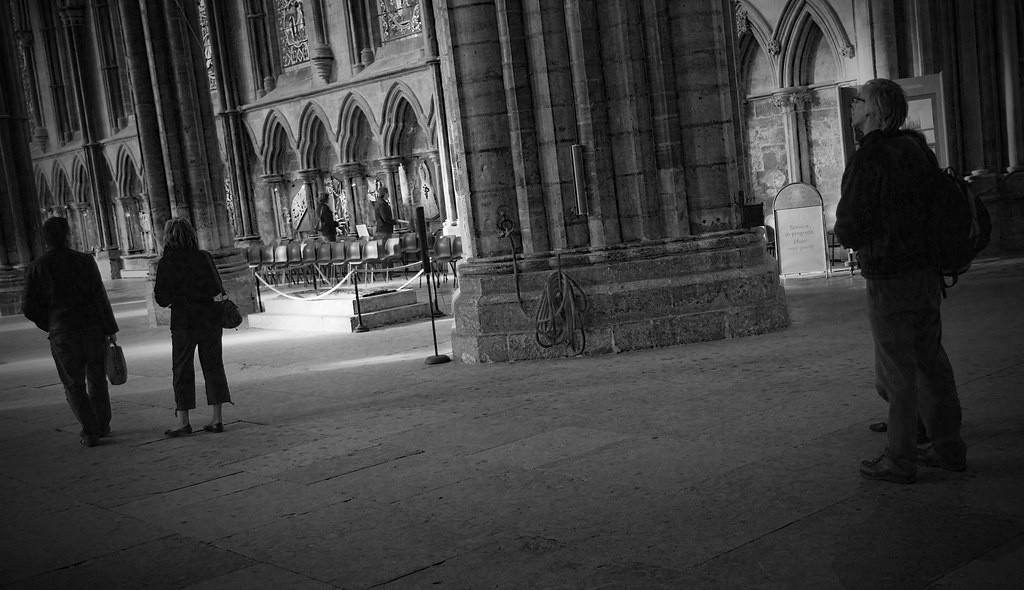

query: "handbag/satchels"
xmin=105 ymin=340 xmax=127 ymax=385
xmin=203 ymin=249 xmax=243 ymax=328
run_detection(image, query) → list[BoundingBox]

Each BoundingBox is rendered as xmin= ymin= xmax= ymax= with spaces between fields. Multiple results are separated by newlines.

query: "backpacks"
xmin=903 ymin=134 xmax=992 ymax=299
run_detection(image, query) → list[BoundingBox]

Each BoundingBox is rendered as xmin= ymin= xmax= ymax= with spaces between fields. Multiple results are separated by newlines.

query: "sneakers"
xmin=860 ymin=447 xmax=918 ymax=485
xmin=919 ymin=444 xmax=968 ymax=471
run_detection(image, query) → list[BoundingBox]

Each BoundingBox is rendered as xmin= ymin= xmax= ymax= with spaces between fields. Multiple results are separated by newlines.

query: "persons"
xmin=375 ymin=187 xmax=398 ymax=251
xmin=22 ymin=216 xmax=119 ymax=446
xmin=154 ymin=218 xmax=232 ymax=437
xmin=834 ymin=79 xmax=966 ymax=485
xmin=316 ymin=193 xmax=339 ymax=242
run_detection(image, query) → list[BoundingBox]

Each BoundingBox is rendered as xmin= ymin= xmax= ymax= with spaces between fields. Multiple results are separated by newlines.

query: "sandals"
xmin=204 ymin=423 xmax=223 ymax=432
xmin=167 ymin=424 xmax=192 ymax=437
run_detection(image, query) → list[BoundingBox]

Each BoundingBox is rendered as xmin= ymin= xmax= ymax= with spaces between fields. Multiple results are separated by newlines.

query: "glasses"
xmin=854 ymin=96 xmax=865 ymax=102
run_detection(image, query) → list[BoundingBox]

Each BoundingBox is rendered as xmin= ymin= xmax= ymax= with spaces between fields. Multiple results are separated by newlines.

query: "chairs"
xmin=824 ymin=203 xmax=842 ymax=267
xmin=242 ymin=228 xmax=464 ymax=289
xmin=765 ymin=215 xmax=776 ymax=257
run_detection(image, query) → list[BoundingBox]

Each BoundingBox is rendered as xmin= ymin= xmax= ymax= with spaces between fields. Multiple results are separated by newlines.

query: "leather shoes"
xmin=870 ymin=421 xmax=888 ymax=433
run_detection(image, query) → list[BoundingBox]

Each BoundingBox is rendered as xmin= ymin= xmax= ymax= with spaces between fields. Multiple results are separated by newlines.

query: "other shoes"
xmin=80 ymin=431 xmax=98 ymax=447
xmin=96 ymin=426 xmax=111 ymax=437
